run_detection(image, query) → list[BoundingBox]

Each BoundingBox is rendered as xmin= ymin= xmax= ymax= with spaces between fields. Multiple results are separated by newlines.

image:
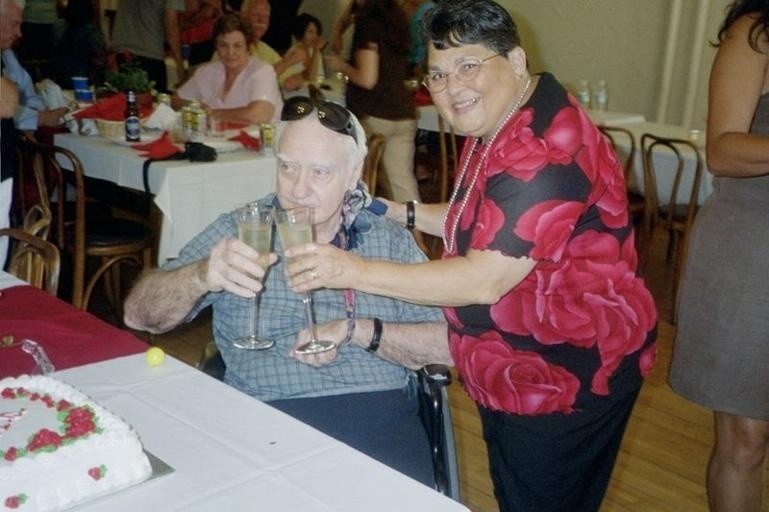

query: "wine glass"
xmin=274 ymin=208 xmax=337 ymax=354
xmin=233 ymin=201 xmax=276 ymax=351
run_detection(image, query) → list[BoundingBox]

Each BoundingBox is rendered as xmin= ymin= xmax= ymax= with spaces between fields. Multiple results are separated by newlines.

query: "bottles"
xmin=126 ymin=90 xmax=141 ymax=141
xmin=577 ymin=79 xmax=590 ymax=107
xmin=595 ymin=81 xmax=608 ymax=120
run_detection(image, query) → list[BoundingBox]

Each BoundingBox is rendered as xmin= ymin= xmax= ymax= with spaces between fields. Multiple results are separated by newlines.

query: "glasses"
xmin=280 ymin=95 xmax=359 ymax=144
xmin=420 ymin=50 xmax=508 ymax=92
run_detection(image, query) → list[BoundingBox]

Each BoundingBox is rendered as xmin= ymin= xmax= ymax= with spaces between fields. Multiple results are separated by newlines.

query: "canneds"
xmin=261 ymin=123 xmax=274 ymax=145
xmin=156 ymin=93 xmax=172 ymax=105
xmin=182 ymin=101 xmax=206 ymax=132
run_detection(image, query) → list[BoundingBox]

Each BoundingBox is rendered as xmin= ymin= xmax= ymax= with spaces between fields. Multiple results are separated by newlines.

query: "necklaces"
xmin=441 ymin=72 xmax=534 ymax=257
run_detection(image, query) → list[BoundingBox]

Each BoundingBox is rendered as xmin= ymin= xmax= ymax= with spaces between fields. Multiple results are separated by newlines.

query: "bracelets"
xmin=363 ymin=316 xmax=385 ymax=356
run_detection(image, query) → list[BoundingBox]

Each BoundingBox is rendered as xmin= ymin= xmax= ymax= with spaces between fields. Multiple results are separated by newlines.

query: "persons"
xmin=282 ymin=0 xmax=659 ymax=511
xmin=1 ymin=0 xmax=443 ymax=255
xmin=666 ymin=1 xmax=769 ymax=512
xmin=120 ymin=94 xmax=456 ymax=492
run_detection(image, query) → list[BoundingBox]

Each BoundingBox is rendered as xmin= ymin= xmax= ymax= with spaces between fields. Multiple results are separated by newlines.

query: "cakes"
xmin=0 ymin=374 xmax=152 ymax=512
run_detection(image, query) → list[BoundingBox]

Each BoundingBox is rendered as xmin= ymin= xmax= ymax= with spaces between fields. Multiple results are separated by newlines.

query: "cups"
xmin=210 ymin=114 xmax=228 ymax=140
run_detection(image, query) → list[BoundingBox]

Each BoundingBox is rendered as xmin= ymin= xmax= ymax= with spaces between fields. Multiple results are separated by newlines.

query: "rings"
xmin=306 ymin=268 xmax=320 ymax=280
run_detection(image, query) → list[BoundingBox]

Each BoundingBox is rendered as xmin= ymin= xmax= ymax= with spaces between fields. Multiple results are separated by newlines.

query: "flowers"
xmin=103 ymin=46 xmax=157 ymax=95
